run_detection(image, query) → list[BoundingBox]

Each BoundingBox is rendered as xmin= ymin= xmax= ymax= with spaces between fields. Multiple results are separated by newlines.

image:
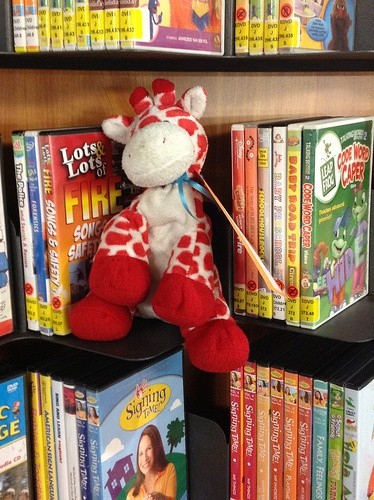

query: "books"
xmin=230 ymin=115 xmax=373 ymax=330
xmin=12 ymin=0 xmax=356 ymax=58
xmin=0 ymin=346 xmax=188 ymax=500
xmin=229 ymin=333 xmax=374 ymax=500
xmin=0 ymin=126 xmax=134 ymax=336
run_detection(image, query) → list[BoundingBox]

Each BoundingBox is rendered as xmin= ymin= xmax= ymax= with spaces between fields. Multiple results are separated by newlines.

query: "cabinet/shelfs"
xmin=0 ymin=48 xmax=374 ymax=394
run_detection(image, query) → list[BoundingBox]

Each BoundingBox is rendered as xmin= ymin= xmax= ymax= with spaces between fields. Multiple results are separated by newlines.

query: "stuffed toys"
xmin=70 ymin=79 xmax=249 ymax=372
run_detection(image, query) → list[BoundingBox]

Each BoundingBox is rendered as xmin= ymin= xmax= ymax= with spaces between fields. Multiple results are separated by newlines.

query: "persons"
xmin=89 ymin=406 xmax=98 ymax=425
xmin=76 ymin=401 xmax=86 ymax=418
xmin=299 ymin=391 xmax=309 ymax=407
xmin=285 ymin=387 xmax=295 ymax=402
xmin=271 ymin=380 xmax=282 ymax=396
xmin=230 ymin=372 xmax=240 ymax=389
xmin=126 ymin=425 xmax=177 ymax=500
xmin=245 ymin=375 xmax=256 ymax=392
xmin=314 ymin=391 xmax=326 ymax=407
xmin=258 ymin=380 xmax=267 ymax=396
